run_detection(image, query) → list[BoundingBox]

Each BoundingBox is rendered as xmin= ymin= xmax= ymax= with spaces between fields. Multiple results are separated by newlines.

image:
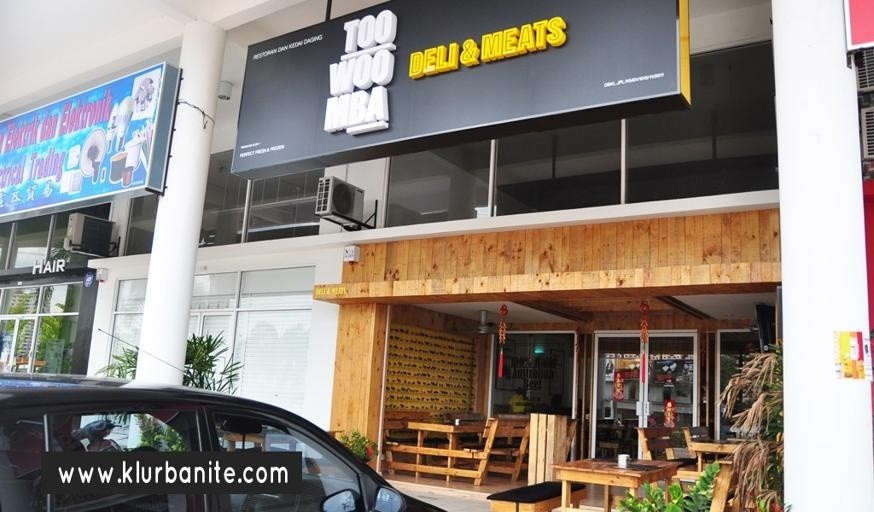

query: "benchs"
xmin=485 ymin=480 xmax=588 ymax=511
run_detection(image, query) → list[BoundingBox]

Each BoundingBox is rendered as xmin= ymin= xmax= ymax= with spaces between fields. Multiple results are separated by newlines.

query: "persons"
xmin=507 ymin=387 xmax=527 ymax=414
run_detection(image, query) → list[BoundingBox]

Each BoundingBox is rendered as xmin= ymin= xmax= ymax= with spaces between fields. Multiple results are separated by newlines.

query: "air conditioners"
xmin=64 ymin=213 xmax=116 ymax=253
xmin=314 ymin=175 xmax=365 ymax=226
xmin=861 ymin=107 xmax=874 ymax=160
xmin=854 ymin=48 xmax=874 ymax=93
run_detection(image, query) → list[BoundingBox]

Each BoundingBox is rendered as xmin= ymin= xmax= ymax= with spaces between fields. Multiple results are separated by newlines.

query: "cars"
xmin=0 ymin=374 xmax=446 ymax=512
xmin=615 ymin=359 xmax=693 ymax=397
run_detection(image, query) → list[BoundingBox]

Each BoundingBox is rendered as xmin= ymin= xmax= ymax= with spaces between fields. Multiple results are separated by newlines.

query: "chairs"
xmin=382 ymin=409 xmax=437 ymax=443
xmin=634 ymin=425 xmax=712 ymax=460
xmin=457 ymin=413 xmax=530 ymax=485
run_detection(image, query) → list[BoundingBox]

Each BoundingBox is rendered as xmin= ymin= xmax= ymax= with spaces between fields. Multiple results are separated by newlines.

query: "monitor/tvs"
xmin=756 ymin=304 xmax=775 ymax=356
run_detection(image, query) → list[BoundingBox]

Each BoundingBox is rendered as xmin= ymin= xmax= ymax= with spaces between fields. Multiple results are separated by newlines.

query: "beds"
xmin=407 ymin=420 xmax=490 ymax=483
